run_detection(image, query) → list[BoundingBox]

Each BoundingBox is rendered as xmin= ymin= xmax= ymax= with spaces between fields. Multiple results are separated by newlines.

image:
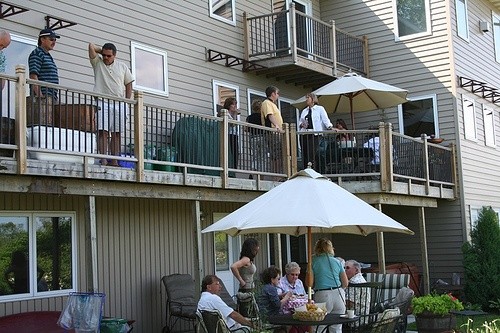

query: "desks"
xmin=450 ymin=311 xmax=489 ymax=330
xmin=339 ymin=148 xmax=375 ymax=172
xmin=266 ymin=313 xmax=361 ymax=333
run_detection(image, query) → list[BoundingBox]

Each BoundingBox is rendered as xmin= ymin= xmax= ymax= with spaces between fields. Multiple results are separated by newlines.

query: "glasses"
xmin=344 ymin=266 xmax=356 ymax=270
xmin=44 ymin=38 xmax=56 ymax=41
xmin=102 ymin=53 xmax=114 ymax=57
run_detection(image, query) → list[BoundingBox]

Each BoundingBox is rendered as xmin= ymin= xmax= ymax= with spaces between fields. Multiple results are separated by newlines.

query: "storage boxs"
xmin=287 ymin=299 xmax=314 ymax=308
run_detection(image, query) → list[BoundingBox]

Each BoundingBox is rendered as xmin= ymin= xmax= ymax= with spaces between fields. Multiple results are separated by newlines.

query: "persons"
xmin=342 ymin=260 xmax=370 ymax=333
xmin=0 ymin=30 xmax=12 ymax=89
xmin=299 ymin=92 xmax=334 ymax=173
xmin=257 ymin=266 xmax=292 ymax=325
xmin=197 ymin=275 xmax=256 ymax=333
xmin=231 ymin=238 xmax=259 ymax=318
xmin=6 ymin=251 xmax=44 ymax=295
xmin=28 ymin=29 xmax=61 ymax=101
xmin=246 ymin=99 xmax=266 ymax=180
xmin=337 ymin=119 xmax=350 ymax=141
xmin=224 ymin=97 xmax=240 ymax=169
xmin=276 ymin=262 xmax=310 ymax=333
xmin=305 ymin=238 xmax=348 ymax=333
xmin=261 ymin=86 xmax=283 ymax=173
xmin=364 ymin=127 xmax=380 ymax=173
xmin=88 ymin=42 xmax=135 ymax=166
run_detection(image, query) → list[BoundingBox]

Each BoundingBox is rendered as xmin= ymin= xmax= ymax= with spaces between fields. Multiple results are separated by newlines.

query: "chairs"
xmin=195 ymin=275 xmax=285 ymax=333
xmin=345 ymin=278 xmax=414 ymax=333
xmin=351 ymin=307 xmax=404 ymax=333
xmin=162 ymin=274 xmax=198 ymax=333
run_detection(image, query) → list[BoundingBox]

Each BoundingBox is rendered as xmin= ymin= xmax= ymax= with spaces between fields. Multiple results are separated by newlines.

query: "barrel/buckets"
xmin=158 ymin=145 xmax=178 ymax=172
xmin=132 ymin=144 xmax=156 ymax=171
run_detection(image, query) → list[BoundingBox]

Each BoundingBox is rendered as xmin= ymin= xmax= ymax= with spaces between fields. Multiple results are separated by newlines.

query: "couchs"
xmin=360 ymin=272 xmax=414 ymax=309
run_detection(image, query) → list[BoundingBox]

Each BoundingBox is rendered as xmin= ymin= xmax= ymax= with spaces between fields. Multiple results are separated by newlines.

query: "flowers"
xmin=411 ymin=291 xmax=464 ymax=317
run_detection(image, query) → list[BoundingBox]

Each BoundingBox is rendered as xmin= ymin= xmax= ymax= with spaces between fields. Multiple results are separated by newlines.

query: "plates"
xmin=340 ymin=314 xmax=359 ymax=318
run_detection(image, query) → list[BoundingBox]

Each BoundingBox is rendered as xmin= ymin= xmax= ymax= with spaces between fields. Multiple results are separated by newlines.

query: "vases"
xmin=416 ymin=309 xmax=456 ymax=333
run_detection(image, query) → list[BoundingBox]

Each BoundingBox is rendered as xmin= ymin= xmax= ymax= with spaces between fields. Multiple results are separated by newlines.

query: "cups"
xmin=347 ymin=310 xmax=354 ymax=319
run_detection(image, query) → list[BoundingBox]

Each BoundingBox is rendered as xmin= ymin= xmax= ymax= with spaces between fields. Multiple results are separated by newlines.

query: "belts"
xmin=315 ymin=287 xmax=340 ymax=292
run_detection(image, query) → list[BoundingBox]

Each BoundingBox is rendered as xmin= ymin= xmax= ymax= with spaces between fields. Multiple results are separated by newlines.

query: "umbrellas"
xmin=201 ymin=162 xmax=414 ymax=262
xmin=290 ymin=69 xmax=409 ymax=130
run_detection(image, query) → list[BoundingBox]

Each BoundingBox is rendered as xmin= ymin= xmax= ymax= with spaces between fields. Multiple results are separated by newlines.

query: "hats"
xmin=38 ymin=29 xmax=60 ymax=38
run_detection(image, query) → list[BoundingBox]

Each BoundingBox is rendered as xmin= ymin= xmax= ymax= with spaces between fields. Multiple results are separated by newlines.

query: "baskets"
xmin=295 ymin=310 xmax=326 ymax=322
